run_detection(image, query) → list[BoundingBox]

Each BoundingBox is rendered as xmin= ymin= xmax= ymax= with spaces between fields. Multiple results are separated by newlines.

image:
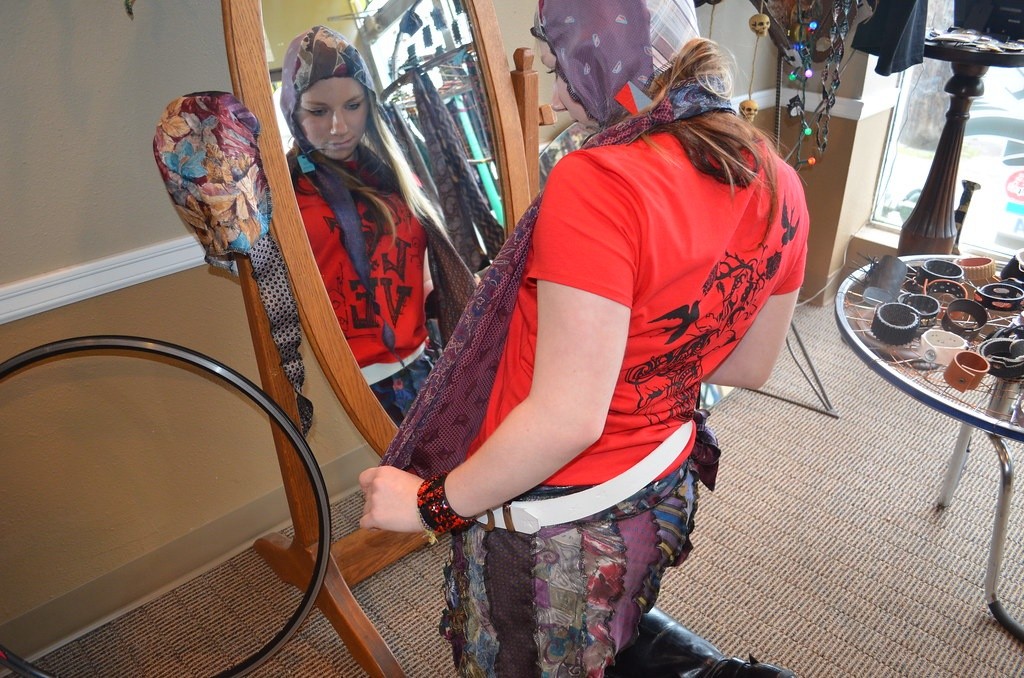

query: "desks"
xmin=897 ymin=28 xmax=1024 ymax=256
xmin=833 ymin=254 xmax=1024 ymax=641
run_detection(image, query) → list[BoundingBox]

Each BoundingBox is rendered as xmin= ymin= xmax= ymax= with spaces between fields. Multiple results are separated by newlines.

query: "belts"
xmin=476 ymin=419 xmax=693 ymax=535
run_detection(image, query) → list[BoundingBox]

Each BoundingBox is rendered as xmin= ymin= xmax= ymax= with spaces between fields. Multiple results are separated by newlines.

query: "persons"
xmin=279 ymin=28 xmax=479 ymax=431
xmin=359 ymin=2 xmax=811 ymax=678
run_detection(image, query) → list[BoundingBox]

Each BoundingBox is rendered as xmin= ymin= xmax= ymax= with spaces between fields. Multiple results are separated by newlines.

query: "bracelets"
xmin=863 ymin=251 xmax=1024 ymax=392
xmin=418 ymin=470 xmax=477 ymax=535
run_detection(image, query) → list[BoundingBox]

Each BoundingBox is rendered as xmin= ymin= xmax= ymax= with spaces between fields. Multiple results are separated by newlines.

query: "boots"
xmin=607 ymin=605 xmax=792 ymax=678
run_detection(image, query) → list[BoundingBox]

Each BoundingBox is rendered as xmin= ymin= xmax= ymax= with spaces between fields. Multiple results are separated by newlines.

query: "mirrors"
xmin=221 ymin=0 xmax=530 ymax=460
